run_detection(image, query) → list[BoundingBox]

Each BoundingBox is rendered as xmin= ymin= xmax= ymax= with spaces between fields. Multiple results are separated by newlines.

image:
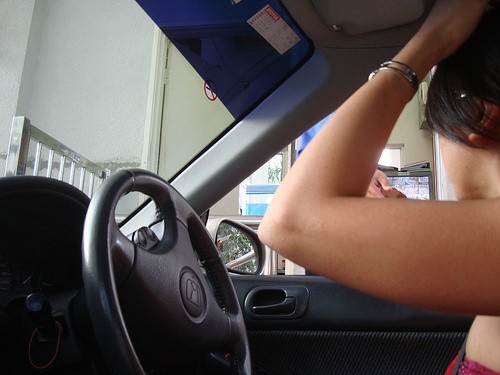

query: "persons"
xmin=259 ymin=0 xmax=499 ymax=375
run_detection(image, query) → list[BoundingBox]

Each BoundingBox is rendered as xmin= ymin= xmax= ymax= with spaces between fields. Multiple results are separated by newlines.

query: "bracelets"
xmin=367 ymin=60 xmax=418 ymax=98
